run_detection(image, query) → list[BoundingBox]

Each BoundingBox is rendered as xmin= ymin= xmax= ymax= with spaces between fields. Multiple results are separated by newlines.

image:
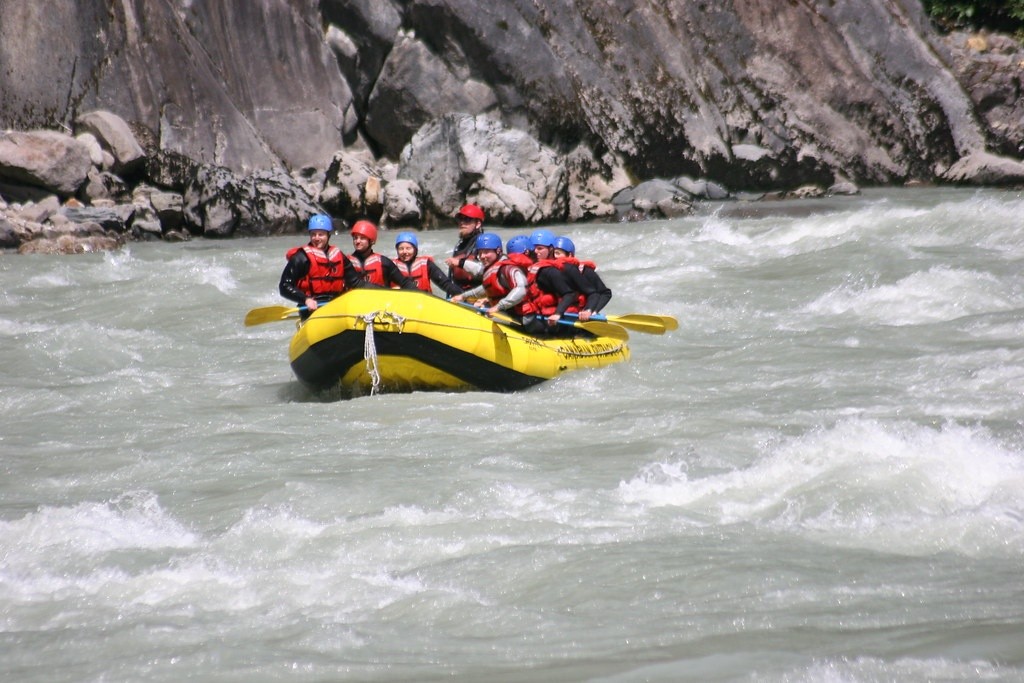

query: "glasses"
xmin=455 ymin=216 xmax=479 ymax=223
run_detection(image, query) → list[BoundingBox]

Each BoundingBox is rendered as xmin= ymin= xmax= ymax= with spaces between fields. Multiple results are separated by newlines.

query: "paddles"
xmin=446 ymin=299 xmax=522 ymax=326
xmin=245 ymin=301 xmax=330 ymax=326
xmin=536 ymin=315 xmax=629 ymax=341
xmin=564 ymin=312 xmax=678 ymax=335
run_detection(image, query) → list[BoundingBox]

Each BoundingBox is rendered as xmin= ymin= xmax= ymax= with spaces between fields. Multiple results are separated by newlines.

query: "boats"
xmin=287 ymin=288 xmax=631 ymax=400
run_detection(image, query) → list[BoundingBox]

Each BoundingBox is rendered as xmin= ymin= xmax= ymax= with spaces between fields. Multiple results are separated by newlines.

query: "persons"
xmin=345 ymin=222 xmax=416 ymax=290
xmin=390 ymin=232 xmax=476 ymax=303
xmin=445 ymin=205 xmax=484 ymax=299
xmin=451 ymin=230 xmax=612 ymax=338
xmin=279 ymin=215 xmax=385 ymax=323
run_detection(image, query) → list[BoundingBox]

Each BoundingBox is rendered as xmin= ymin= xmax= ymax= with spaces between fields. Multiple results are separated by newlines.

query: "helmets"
xmin=475 ymin=232 xmax=503 ymax=254
xmin=506 ymin=234 xmax=535 ymax=255
xmin=454 ymin=205 xmax=485 ymax=223
xmin=308 ymin=215 xmax=333 ymax=232
xmin=395 ymin=231 xmax=418 ymax=255
xmin=530 ymin=229 xmax=556 ymax=247
xmin=351 ymin=220 xmax=377 ymax=243
xmin=553 ymin=236 xmax=575 ymax=256
